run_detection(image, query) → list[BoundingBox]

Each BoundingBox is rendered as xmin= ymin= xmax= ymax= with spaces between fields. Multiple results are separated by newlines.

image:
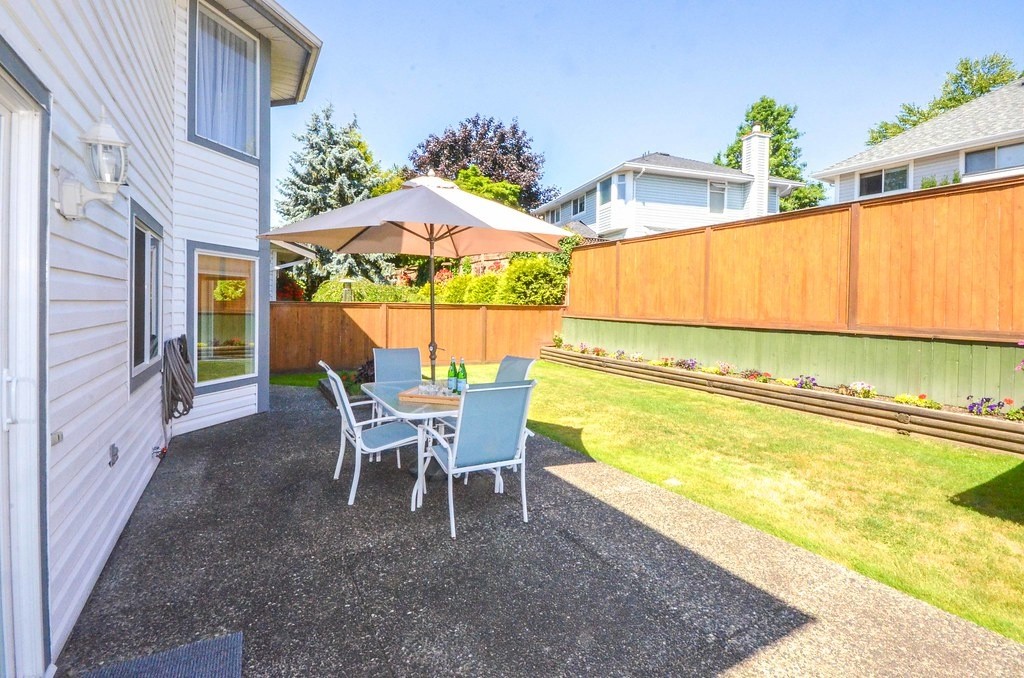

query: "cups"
xmin=419 ymin=383 xmax=453 ymax=396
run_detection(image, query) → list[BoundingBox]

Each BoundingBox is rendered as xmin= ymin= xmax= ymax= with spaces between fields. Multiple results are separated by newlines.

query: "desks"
xmin=362 ymin=376 xmax=494 ymax=494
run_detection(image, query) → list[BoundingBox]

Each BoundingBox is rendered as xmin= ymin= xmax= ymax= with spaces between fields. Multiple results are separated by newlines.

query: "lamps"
xmin=57 ymin=106 xmax=136 ymax=219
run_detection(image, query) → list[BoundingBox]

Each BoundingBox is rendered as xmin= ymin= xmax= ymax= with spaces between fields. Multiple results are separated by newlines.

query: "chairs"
xmin=316 ymin=362 xmax=430 ymax=506
xmin=412 ymin=378 xmax=538 ymax=539
xmin=368 ymin=346 xmax=425 ymax=462
xmin=493 ymin=353 xmax=537 ymax=473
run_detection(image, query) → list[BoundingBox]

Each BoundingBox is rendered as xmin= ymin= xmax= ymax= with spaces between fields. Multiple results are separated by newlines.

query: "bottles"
xmin=457 ymin=358 xmax=467 ymax=395
xmin=448 ymin=356 xmax=457 ymax=393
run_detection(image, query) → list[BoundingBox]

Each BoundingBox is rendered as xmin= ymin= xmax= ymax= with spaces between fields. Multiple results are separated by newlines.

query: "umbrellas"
xmin=256 ymin=168 xmax=576 ymax=445
